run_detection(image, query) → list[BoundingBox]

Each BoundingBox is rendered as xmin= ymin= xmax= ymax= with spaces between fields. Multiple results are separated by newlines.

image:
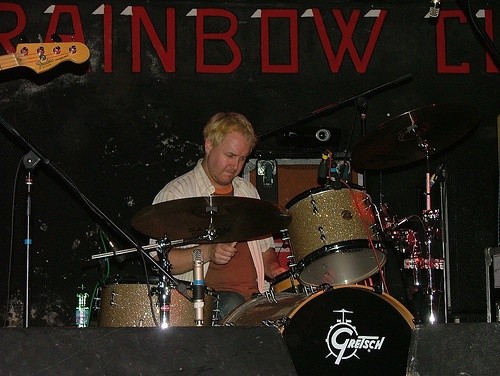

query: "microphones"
xmin=192 ymin=249 xmax=205 ymax=327
xmin=317 ymin=151 xmax=331 ymax=185
xmin=357 ymin=98 xmax=367 ymax=137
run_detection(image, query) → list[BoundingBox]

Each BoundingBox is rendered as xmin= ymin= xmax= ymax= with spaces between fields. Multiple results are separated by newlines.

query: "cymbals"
xmin=351 ymin=102 xmax=480 ymax=169
xmin=131 ymin=195 xmax=292 ymax=245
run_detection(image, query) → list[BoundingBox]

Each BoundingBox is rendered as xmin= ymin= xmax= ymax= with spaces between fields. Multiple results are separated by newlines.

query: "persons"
xmin=148 ymin=112 xmax=285 ymax=324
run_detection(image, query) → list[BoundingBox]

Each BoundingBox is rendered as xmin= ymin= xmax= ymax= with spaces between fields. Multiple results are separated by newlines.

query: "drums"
xmin=98 ymin=278 xmax=216 ymax=327
xmin=219 ymin=284 xmax=416 ymax=376
xmin=284 ymin=182 xmax=387 ymax=287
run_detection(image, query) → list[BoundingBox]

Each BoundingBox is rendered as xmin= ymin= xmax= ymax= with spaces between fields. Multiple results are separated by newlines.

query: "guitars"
xmin=0 ymin=42 xmax=91 ymax=75
